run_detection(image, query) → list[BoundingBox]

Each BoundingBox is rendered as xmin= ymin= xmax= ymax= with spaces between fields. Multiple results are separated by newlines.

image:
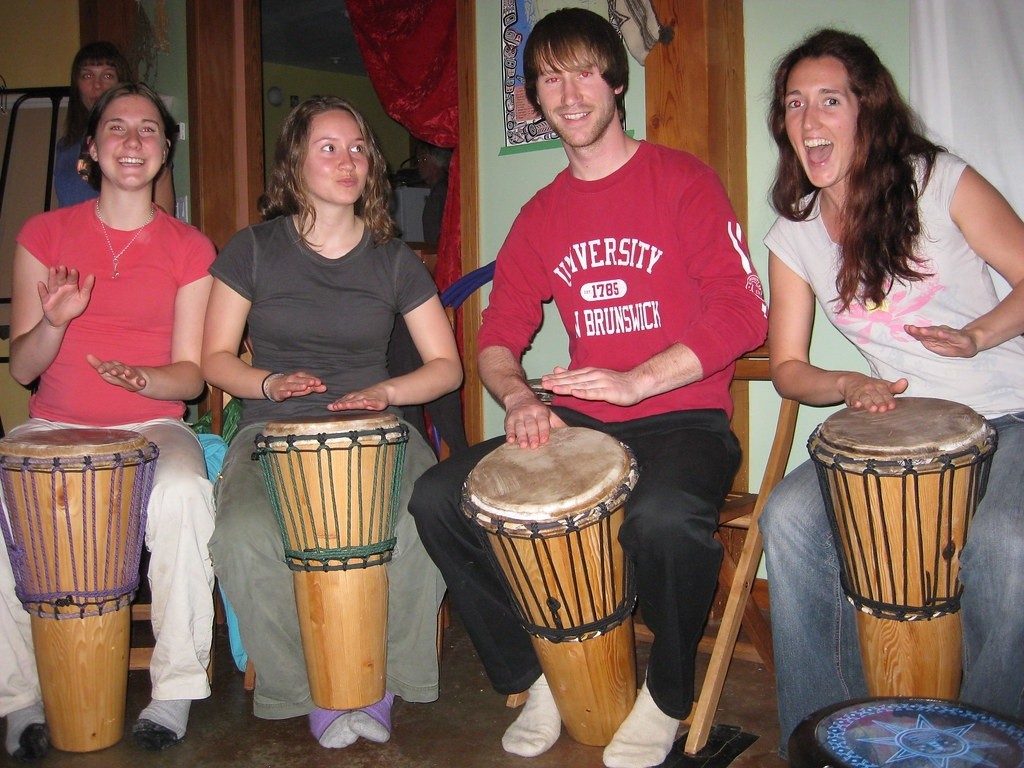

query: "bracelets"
xmin=262 ymin=371 xmax=284 ymax=403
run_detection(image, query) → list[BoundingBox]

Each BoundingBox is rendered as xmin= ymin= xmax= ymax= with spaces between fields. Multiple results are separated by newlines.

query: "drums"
xmin=786 ymin=696 xmax=1024 ymax=768
xmin=0 ymin=425 xmax=159 ymax=754
xmin=805 ymin=387 xmax=1000 ymax=704
xmin=250 ymin=409 xmax=412 ymax=715
xmin=464 ymin=422 xmax=644 ymax=749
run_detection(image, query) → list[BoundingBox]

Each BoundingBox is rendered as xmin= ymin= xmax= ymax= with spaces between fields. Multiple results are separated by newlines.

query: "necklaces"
xmin=94 ymin=196 xmax=154 ymax=279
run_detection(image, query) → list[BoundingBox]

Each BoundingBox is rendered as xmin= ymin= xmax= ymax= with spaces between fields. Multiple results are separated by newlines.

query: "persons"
xmin=753 ymin=23 xmax=1022 ymax=756
xmin=0 ymin=80 xmax=217 ymax=745
xmin=407 ymin=8 xmax=772 ymax=765
xmin=54 ymin=43 xmax=177 ymax=218
xmin=200 ymin=92 xmax=464 ymax=747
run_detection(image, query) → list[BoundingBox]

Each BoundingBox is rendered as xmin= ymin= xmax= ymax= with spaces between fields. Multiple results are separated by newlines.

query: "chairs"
xmin=612 ymin=318 xmax=811 ymax=758
